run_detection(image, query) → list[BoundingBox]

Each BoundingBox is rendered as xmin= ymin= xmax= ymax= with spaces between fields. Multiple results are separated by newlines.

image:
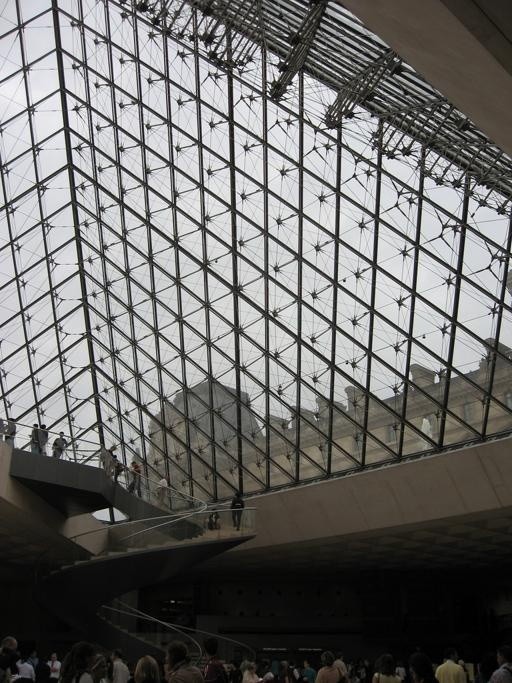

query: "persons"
xmin=0 ymin=637 xmax=512 ymax=681
xmin=230 ymin=489 xmax=244 ymax=530
xmin=1 ymin=418 xmax=141 ymax=500
xmin=208 ymin=509 xmax=221 ymax=528
